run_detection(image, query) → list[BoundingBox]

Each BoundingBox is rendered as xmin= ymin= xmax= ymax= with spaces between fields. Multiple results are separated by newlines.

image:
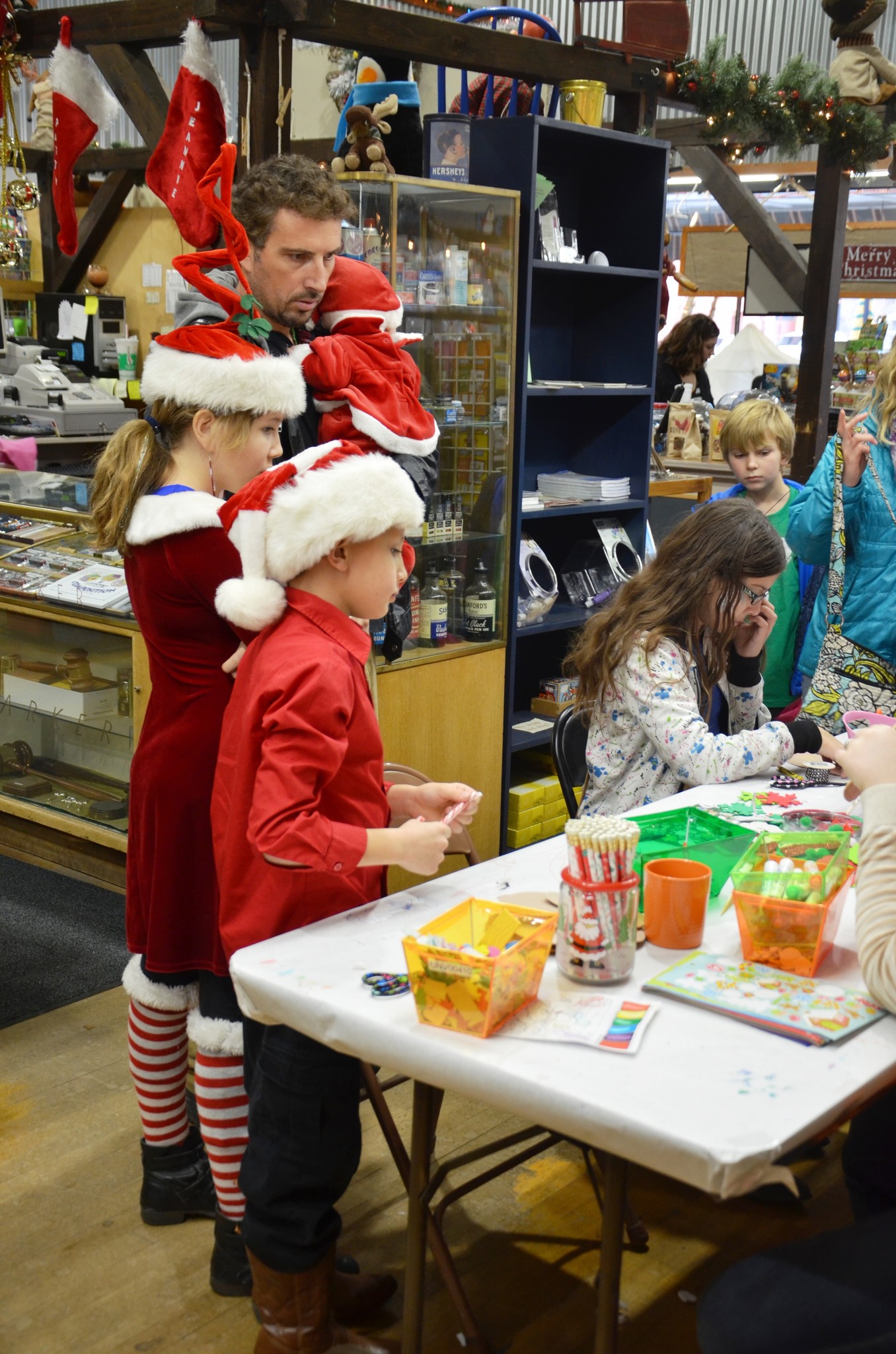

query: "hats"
xmin=214 ymin=439 xmax=428 ymax=630
xmin=318 ymin=256 xmax=404 ymax=330
xmin=140 ymin=144 xmax=306 ymax=418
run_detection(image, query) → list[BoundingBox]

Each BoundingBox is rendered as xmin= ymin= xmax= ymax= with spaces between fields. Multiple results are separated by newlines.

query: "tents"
xmin=701 ymin=323 xmax=809 ymax=405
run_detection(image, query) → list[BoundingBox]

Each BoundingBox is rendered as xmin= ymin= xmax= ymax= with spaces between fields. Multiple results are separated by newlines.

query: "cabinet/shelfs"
xmin=333 ymin=170 xmax=520 ymax=892
xmin=0 ymin=467 xmax=505 ymax=902
xmin=468 ymin=115 xmax=672 ymax=856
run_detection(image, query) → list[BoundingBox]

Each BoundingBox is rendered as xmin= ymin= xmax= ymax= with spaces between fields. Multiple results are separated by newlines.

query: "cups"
xmin=116 ymin=669 xmax=133 ymax=715
xmin=114 ymin=338 xmax=139 ymax=380
xmin=644 ymin=857 xmax=712 ymax=948
xmin=556 ymin=867 xmax=640 ymax=985
xmin=602 ymin=94 xmax=615 ymax=130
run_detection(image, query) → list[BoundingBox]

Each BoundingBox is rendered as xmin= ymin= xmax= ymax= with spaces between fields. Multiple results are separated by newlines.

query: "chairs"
xmin=551 ymin=700 xmax=595 ymax=820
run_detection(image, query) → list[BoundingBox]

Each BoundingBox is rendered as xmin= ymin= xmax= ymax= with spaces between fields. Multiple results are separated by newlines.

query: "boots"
xmin=211 ymin=1206 xmax=361 ymax=1297
xmin=326 ymin=1254 xmax=398 ymax=1329
xmin=248 ymin=1249 xmax=403 ymax=1354
xmin=140 ymin=1130 xmax=221 ymax=1225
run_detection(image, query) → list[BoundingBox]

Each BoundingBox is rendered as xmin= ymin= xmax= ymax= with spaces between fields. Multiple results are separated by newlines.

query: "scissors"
xmin=361 ymin=972 xmax=410 ymax=997
xmin=770 ymin=775 xmax=847 ymax=789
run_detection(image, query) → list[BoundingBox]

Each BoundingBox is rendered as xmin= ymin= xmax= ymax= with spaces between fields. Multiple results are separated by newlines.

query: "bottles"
xmin=372 ymin=558 xmax=497 ymax=657
xmin=491 ymin=401 xmax=508 ymax=421
xmin=419 ymin=392 xmax=465 ymax=425
xmin=364 ymin=218 xmax=381 ymax=272
xmin=467 ymin=274 xmax=483 ymax=306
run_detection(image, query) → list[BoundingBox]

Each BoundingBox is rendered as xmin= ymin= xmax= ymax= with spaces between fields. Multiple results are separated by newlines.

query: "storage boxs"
xmin=2 ymin=661 xmax=118 ymax=720
xmin=505 ymin=775 xmax=582 ymax=849
xmin=402 ymin=897 xmax=558 ymax=1038
xmin=622 ymin=806 xmax=856 ymax=978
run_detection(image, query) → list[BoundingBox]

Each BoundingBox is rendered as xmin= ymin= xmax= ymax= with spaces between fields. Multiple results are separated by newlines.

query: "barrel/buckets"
xmin=559 ymin=79 xmax=607 ymax=129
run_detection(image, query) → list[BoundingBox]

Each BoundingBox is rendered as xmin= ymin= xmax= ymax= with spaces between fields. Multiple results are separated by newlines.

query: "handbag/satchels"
xmin=795 ymin=627 xmax=896 ymax=737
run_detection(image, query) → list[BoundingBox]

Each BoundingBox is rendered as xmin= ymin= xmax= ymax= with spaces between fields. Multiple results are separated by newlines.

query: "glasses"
xmin=732 ymin=578 xmax=770 ymax=606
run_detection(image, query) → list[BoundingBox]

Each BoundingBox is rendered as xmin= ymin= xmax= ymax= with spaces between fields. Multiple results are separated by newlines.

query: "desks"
xmin=228 ymin=774 xmax=896 ymax=1354
xmin=648 ymin=470 xmax=712 ymax=504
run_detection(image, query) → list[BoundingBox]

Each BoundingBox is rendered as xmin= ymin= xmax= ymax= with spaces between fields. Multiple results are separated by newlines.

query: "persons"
xmin=563 ymin=321 xmax=896 ymax=1353
xmin=83 ymin=151 xmax=484 ymax=1354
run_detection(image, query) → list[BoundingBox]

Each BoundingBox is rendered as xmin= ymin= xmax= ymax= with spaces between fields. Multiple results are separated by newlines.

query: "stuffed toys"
xmin=318 ymin=45 xmax=429 ymax=179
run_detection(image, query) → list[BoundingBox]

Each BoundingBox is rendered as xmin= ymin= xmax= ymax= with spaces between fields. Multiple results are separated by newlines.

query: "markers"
xmin=761 ymin=857 xmax=841 ymax=905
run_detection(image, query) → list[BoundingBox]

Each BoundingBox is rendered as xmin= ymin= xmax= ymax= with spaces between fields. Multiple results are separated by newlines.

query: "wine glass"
xmin=86 ymin=264 xmax=109 ymax=296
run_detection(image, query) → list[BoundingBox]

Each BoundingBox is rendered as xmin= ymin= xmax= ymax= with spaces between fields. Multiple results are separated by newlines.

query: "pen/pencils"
xmin=777 ymin=765 xmax=804 ymax=780
xmin=564 ymin=814 xmax=641 ymax=981
